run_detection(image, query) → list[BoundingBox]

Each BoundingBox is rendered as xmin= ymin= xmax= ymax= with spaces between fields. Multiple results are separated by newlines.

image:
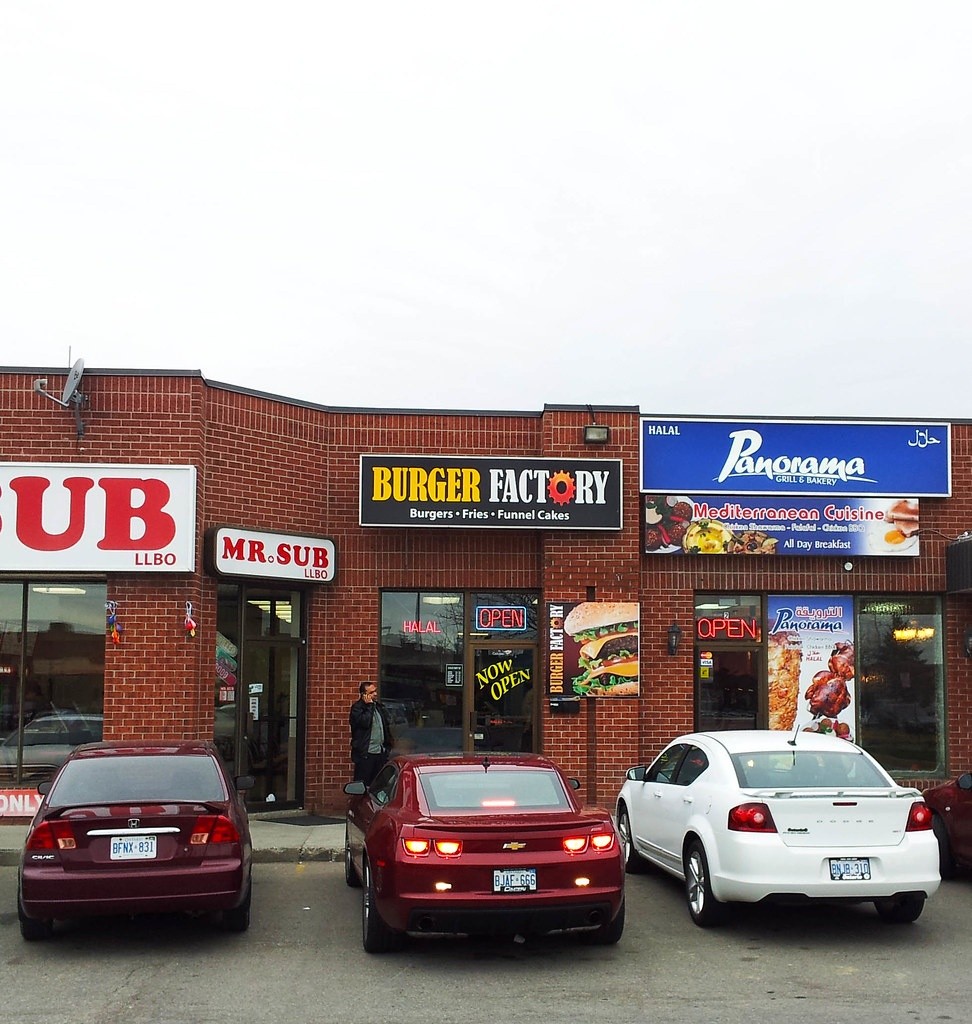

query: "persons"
xmin=349 ymin=682 xmax=395 ymax=786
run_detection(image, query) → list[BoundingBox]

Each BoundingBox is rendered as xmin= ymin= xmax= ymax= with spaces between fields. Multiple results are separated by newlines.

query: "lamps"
xmin=582 ymin=405 xmax=609 ymax=444
xmin=667 ymin=623 xmax=681 ymax=656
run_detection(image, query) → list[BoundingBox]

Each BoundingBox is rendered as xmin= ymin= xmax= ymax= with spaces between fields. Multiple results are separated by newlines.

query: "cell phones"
xmin=363 ymin=693 xmax=369 ymax=699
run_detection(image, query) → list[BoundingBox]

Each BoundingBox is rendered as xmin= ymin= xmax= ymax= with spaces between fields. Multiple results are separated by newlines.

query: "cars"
xmin=0 ymin=713 xmax=104 ymax=782
xmin=921 ymin=769 xmax=972 ymax=883
xmin=16 ymin=740 xmax=255 ymax=942
xmin=615 ymin=730 xmax=942 ymax=930
xmin=216 ymin=703 xmax=237 ymax=761
xmin=343 ymin=752 xmax=626 ymax=956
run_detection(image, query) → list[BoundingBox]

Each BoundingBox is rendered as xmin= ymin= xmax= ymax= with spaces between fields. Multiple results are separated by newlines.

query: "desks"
xmin=401 ymin=725 xmax=523 ymax=753
